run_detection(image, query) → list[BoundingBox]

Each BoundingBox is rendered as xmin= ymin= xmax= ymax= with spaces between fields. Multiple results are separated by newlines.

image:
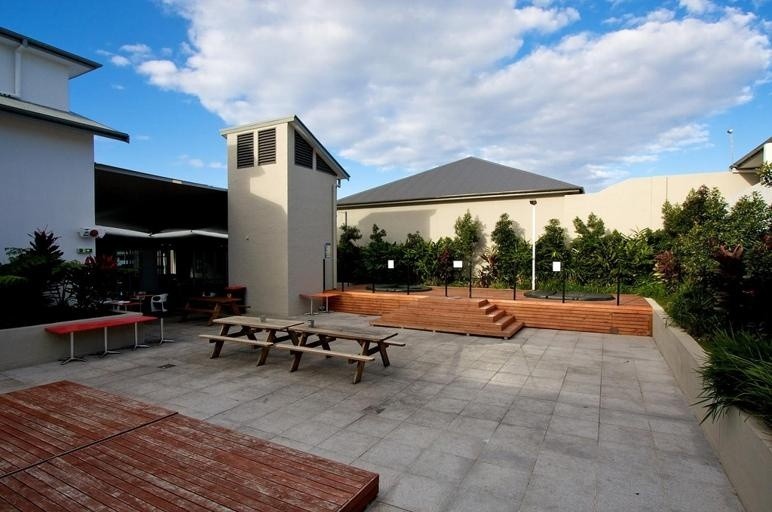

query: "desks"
xmin=300 ymin=292 xmax=341 ymax=316
xmin=44 ymin=315 xmax=158 ymax=365
xmin=1 ymin=380 xmax=178 ymax=492
xmin=208 ymin=316 xmax=306 ymax=366
xmin=289 ymin=323 xmax=398 ymax=384
xmin=176 ymin=295 xmax=242 ymax=326
xmin=0 ymin=413 xmax=378 ymax=512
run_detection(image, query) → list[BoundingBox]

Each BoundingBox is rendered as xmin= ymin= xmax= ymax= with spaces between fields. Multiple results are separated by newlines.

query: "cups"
xmin=307 ymin=319 xmax=315 ymax=329
xmin=259 ymin=313 xmax=268 ymax=323
xmin=226 ymin=293 xmax=232 ymax=299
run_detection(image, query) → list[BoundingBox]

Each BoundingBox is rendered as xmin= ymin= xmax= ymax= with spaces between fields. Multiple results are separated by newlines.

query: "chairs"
xmin=99 ymin=290 xmax=170 ymax=312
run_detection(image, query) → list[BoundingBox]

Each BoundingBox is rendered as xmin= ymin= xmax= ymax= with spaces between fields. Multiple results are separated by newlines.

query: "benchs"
xmin=223 ymin=303 xmax=251 ymax=308
xmin=382 ymin=340 xmax=407 ymax=346
xmin=275 ymin=343 xmax=376 ymax=364
xmin=197 ymin=335 xmax=273 ymax=349
xmin=180 ymin=308 xmax=219 ymax=314
